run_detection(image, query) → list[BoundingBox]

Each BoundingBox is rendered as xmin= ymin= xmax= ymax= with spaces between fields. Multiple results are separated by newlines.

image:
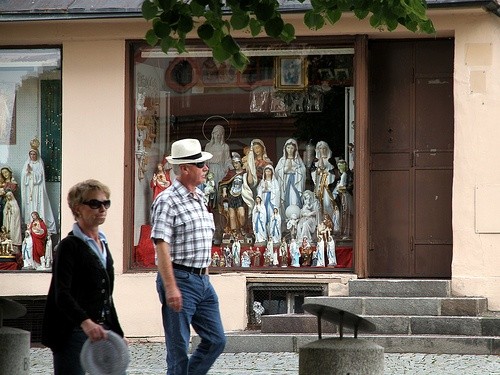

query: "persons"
xmin=150 ymin=125 xmax=353 ymax=267
xmin=41 ymin=179 xmax=129 ymax=375
xmin=0 ymin=138 xmax=57 ymax=270
xmin=151 ymin=139 xmax=226 ymax=375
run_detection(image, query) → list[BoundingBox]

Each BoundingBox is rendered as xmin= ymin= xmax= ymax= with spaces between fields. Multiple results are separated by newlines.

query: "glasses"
xmin=80 ymin=199 xmax=110 ymax=209
xmin=186 ymin=161 xmax=204 ymax=168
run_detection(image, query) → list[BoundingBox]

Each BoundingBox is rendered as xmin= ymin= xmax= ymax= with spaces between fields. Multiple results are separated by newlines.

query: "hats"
xmin=165 ymin=139 xmax=213 ymax=165
xmin=80 ymin=330 xmax=129 ymax=375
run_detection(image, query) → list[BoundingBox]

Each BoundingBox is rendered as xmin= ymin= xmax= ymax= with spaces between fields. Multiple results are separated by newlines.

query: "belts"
xmin=172 ymin=262 xmax=206 ymax=275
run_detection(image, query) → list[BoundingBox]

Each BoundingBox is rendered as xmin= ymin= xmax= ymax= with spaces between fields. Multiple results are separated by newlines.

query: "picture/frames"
xmin=275 ymin=56 xmax=308 ymax=91
